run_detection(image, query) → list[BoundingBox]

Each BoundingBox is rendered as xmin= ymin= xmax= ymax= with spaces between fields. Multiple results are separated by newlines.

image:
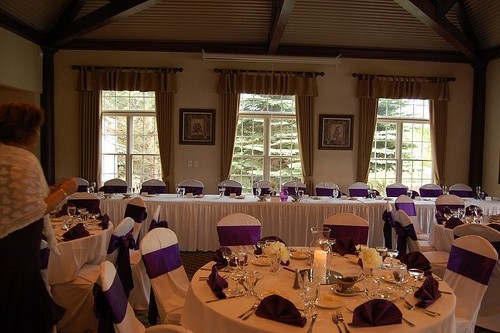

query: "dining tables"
xmin=46 ymin=214 xmax=114 ymax=285
xmin=93 ymin=191 xmax=500 ymax=252
xmin=429 ymin=215 xmax=500 ymax=252
xmin=180 ymin=245 xmax=457 ymax=333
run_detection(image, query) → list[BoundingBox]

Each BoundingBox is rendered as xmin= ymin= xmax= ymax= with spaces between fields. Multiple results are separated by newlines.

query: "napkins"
xmin=415 ymin=276 xmax=441 ymax=308
xmin=255 ymin=294 xmax=307 ymax=328
xmin=96 ymin=213 xmax=109 ymax=230
xmin=207 ymin=265 xmax=228 ymax=299
xmin=396 ymin=251 xmax=432 ymax=276
xmin=63 ymin=222 xmax=90 ymax=241
xmin=332 ymin=238 xmax=359 ymax=255
xmin=346 ymin=298 xmax=402 ymax=328
xmin=253 ymin=236 xmax=286 ymax=255
xmin=212 ymin=249 xmax=229 ymax=270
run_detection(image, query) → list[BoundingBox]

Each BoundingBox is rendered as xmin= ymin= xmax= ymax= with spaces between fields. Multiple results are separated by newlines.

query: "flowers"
xmin=360 ymin=247 xmax=383 ymax=271
xmin=265 ymin=242 xmax=290 ymax=263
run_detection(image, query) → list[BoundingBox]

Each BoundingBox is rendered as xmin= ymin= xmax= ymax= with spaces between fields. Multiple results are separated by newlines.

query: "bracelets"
xmin=59 ymin=189 xmax=67 ymax=196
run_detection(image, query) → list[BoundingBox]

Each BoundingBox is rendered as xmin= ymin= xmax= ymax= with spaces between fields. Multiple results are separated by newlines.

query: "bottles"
xmin=309 ymin=226 xmax=332 ymax=285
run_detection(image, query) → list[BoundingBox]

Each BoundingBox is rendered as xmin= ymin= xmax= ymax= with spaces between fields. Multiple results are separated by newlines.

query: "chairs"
xmin=39 ymin=178 xmax=500 ymax=333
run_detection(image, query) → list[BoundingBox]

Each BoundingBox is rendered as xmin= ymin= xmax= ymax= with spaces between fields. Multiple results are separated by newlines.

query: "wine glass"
xmin=294 ymin=184 xmax=304 ymax=201
xmin=475 ymin=186 xmax=484 ymax=201
xmin=367 ymin=246 xmax=424 ymax=309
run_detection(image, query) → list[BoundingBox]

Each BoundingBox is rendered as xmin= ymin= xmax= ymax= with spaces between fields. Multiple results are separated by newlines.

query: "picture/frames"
xmin=179 ymin=108 xmax=216 ymax=145
xmin=318 ymin=114 xmax=354 ymax=151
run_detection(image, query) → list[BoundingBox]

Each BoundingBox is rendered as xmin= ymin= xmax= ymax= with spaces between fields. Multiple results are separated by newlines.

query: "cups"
xmin=299 ymin=285 xmax=318 ymax=318
xmin=229 ymin=194 xmax=236 ymax=200
xmin=331 ymin=269 xmax=366 ymax=295
xmin=333 ymin=189 xmax=339 ymax=201
xmin=264 ymin=194 xmax=270 ymax=202
xmin=222 ymin=240 xmax=275 ymax=297
xmin=457 ymin=206 xmax=483 ymax=225
xmin=254 ymin=189 xmax=261 ymax=196
xmin=176 ymin=188 xmax=186 ymax=197
xmin=368 ymin=186 xmax=447 ymax=199
xmin=68 ymin=205 xmax=89 ymax=222
xmin=87 ymin=182 xmax=96 ymax=193
xmin=218 ymin=187 xmax=225 ymax=198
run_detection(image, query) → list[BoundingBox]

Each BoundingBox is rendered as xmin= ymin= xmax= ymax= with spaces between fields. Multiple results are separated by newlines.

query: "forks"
xmin=237 ymin=301 xmax=259 ymax=320
xmin=403 ymin=299 xmax=441 ymax=318
xmin=331 ymin=311 xmax=350 ymax=333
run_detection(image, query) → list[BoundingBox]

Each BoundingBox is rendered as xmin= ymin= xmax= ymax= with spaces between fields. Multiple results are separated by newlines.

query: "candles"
xmin=313 ymin=250 xmax=327 ymax=285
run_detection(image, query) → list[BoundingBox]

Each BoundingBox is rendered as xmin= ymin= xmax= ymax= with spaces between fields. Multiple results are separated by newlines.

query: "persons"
xmin=0 ymin=102 xmax=79 ymax=333
